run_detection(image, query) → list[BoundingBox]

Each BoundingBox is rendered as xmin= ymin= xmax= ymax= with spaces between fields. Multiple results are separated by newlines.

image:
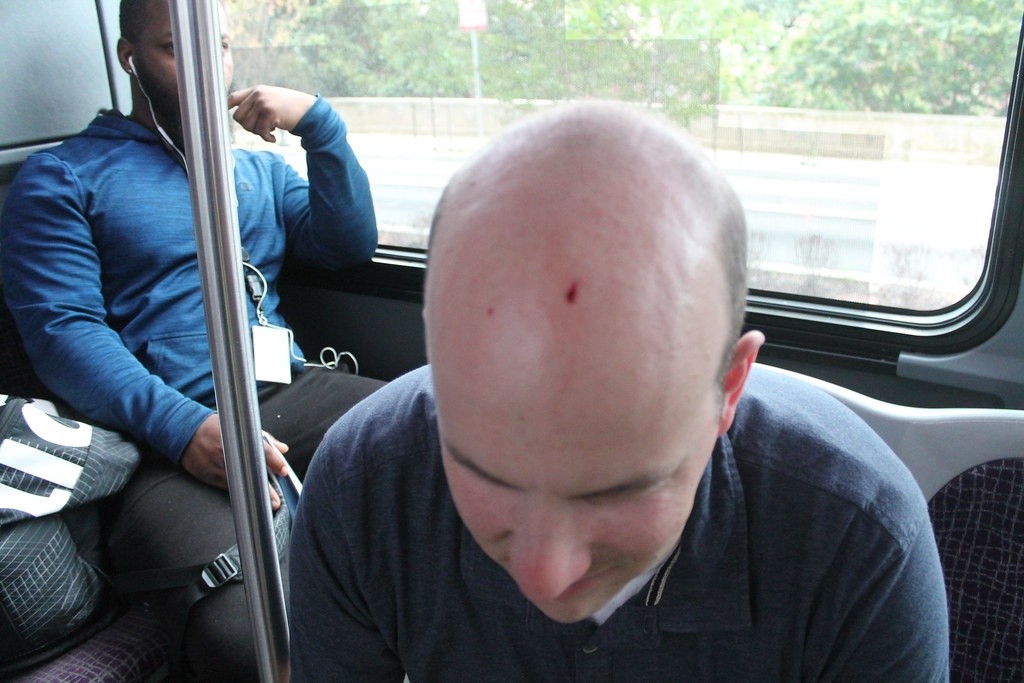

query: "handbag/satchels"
xmin=0 ymin=394 xmax=141 ymax=675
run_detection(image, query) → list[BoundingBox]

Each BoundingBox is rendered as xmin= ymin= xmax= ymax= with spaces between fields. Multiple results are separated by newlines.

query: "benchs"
xmin=739 ymin=362 xmax=1023 ymax=682
xmin=0 ymin=0 xmax=293 ymax=683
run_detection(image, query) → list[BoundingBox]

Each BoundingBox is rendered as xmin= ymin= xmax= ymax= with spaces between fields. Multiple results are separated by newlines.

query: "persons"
xmin=288 ymin=99 xmax=950 ymax=683
xmin=0 ymin=0 xmax=388 ymax=683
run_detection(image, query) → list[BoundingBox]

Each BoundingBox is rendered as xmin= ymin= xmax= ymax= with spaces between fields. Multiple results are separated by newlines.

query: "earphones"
xmin=127 ymin=55 xmax=137 ymax=76
